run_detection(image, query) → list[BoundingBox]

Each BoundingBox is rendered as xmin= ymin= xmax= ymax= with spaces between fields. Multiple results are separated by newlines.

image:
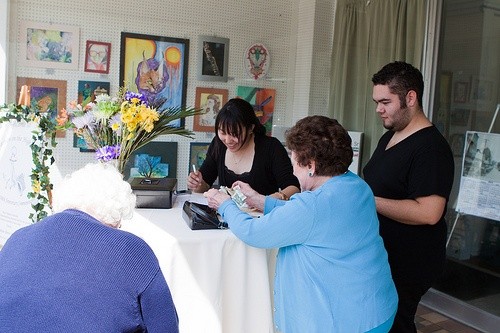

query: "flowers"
xmin=52 ymin=88 xmax=204 ymax=163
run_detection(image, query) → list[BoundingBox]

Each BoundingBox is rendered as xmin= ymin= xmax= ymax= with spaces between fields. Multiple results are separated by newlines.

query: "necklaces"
xmin=228 ymin=138 xmax=250 ymax=166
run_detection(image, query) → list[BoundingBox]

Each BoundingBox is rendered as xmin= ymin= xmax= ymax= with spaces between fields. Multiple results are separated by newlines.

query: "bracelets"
xmin=275 ymin=191 xmax=289 ymax=201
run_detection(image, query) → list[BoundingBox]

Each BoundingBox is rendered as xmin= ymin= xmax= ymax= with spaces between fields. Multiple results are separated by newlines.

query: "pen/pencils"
xmin=193 ymin=164 xmax=196 ymax=171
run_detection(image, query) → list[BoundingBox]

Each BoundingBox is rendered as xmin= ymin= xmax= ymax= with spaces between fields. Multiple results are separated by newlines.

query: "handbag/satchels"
xmin=182 ymin=201 xmax=228 ymax=231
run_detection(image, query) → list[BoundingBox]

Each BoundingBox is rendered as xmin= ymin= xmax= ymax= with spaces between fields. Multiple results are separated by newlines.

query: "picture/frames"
xmin=85 ymin=39 xmax=112 ymax=74
xmin=198 ymin=35 xmax=229 ymax=81
xmin=119 ymin=33 xmax=192 ymax=136
xmin=18 ymin=19 xmax=80 ymax=70
xmin=194 ymin=86 xmax=230 ymax=130
xmin=16 ymin=75 xmax=67 ymax=138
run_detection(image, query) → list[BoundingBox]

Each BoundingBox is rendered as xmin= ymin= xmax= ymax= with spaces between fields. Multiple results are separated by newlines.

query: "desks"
xmin=118 ymin=193 xmax=281 ymax=333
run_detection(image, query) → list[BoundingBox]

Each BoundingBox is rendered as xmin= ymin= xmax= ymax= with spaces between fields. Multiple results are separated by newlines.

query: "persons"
xmin=0 ymin=162 xmax=179 ymax=333
xmin=362 ymin=61 xmax=456 ymax=333
xmin=203 ymin=116 xmax=397 ymax=333
xmin=186 ymin=99 xmax=302 ymax=212
